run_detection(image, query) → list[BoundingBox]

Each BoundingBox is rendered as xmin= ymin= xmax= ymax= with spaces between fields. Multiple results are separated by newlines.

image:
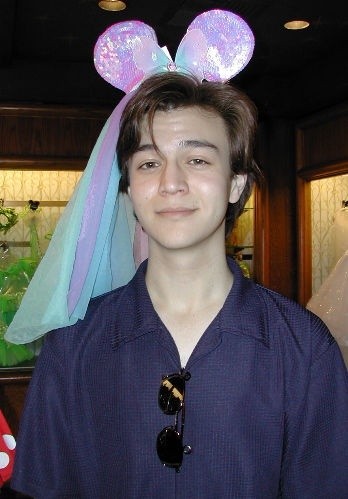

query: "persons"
xmin=7 ymin=70 xmax=348 ymax=499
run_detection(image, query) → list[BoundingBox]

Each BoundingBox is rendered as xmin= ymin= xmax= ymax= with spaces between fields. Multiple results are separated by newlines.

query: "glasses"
xmin=156 ymin=372 xmax=186 ymax=471
